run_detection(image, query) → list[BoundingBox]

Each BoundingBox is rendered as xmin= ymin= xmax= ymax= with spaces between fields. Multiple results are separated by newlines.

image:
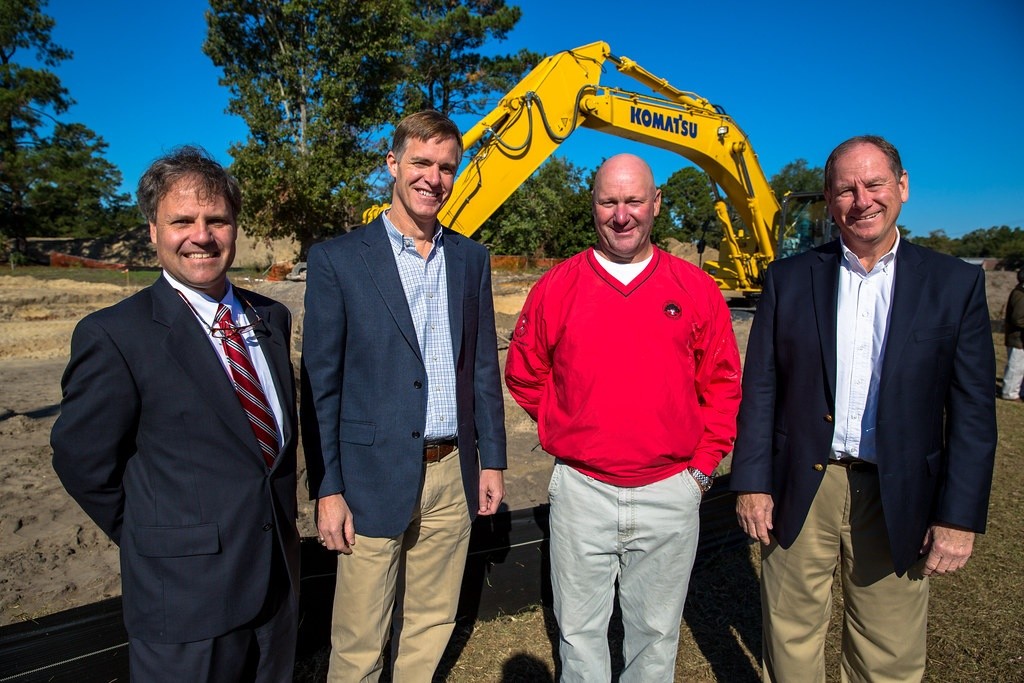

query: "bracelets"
xmin=690 ymin=467 xmax=713 ymax=491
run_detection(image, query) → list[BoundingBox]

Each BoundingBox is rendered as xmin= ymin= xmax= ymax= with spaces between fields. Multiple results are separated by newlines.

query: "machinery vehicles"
xmin=363 ymin=40 xmax=839 ymax=304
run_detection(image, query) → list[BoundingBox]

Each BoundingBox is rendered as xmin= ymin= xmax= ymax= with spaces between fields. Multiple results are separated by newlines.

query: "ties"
xmin=214 ymin=304 xmax=279 ymax=470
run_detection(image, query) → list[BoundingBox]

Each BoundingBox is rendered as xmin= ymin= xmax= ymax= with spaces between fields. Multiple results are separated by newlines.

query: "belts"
xmin=828 ymin=457 xmax=877 ymax=473
xmin=424 ymin=437 xmax=458 ymax=464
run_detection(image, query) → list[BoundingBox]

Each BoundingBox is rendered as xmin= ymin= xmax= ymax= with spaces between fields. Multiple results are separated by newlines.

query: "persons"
xmin=1001 ymin=267 xmax=1024 ymax=400
xmin=504 ymin=153 xmax=742 ymax=682
xmin=50 ymin=146 xmax=301 ymax=683
xmin=300 ymin=109 xmax=507 ymax=683
xmin=729 ymin=134 xmax=997 ymax=683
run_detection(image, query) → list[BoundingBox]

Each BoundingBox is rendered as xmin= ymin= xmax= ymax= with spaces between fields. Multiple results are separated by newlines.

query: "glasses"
xmin=175 ymin=289 xmax=264 ymax=338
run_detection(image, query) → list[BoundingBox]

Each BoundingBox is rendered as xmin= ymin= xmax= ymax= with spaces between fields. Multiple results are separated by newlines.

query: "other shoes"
xmin=1001 ymin=395 xmax=1024 ymax=403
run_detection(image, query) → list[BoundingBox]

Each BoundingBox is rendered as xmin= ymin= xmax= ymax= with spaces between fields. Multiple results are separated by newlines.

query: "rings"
xmin=318 ymin=538 xmax=324 ymax=542
